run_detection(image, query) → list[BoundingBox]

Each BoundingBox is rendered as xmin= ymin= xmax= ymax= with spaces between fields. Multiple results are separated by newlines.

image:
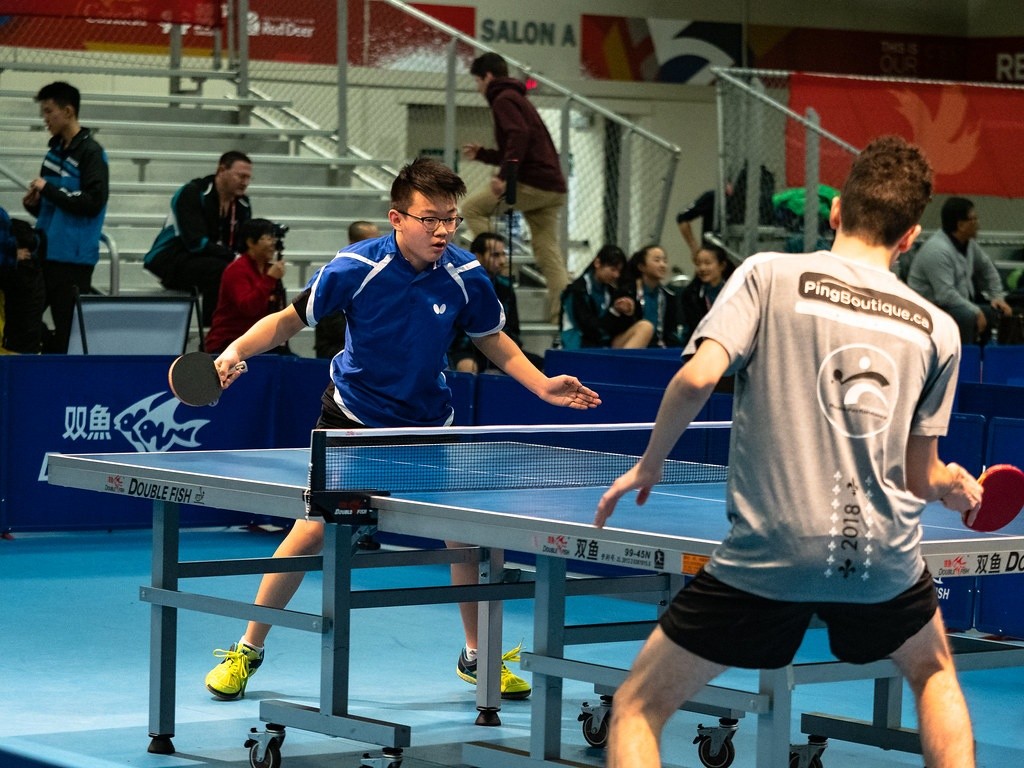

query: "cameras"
xmin=272 ymin=223 xmax=289 ymax=239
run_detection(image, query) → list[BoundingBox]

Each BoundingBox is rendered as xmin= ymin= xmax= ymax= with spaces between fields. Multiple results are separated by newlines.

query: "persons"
xmin=450 ymin=231 xmax=546 ymax=377
xmin=907 ymin=196 xmax=1012 ymax=344
xmin=0 ymin=81 xmax=110 ymax=354
xmin=676 ymin=190 xmax=730 ymax=266
xmin=314 ymin=221 xmax=381 ymax=360
xmin=593 ymin=131 xmax=984 ymax=768
xmin=461 ymin=51 xmax=573 ymax=325
xmin=571 ymin=245 xmax=736 ymax=350
xmin=205 ymin=155 xmax=602 ymax=699
xmin=204 ymin=217 xmax=286 ymax=352
xmin=143 ymin=150 xmax=252 ymax=331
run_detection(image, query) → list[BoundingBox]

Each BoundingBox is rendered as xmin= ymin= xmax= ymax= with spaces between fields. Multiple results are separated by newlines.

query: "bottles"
xmin=988 ymin=328 xmax=998 ymax=342
xmin=507 ymin=211 xmax=521 ymax=238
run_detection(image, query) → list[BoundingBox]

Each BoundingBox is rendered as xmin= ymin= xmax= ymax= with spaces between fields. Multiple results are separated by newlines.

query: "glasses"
xmin=398 ymin=209 xmax=464 ymax=233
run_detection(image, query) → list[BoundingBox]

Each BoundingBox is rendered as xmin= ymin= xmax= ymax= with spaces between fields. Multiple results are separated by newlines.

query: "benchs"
xmin=728 ymin=223 xmax=1024 ymax=330
xmin=0 ymin=58 xmax=559 ymax=355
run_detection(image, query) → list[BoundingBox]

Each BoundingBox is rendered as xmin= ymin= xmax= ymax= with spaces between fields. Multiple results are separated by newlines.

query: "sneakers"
xmin=205 ymin=644 xmax=264 ymax=700
xmin=455 ymin=647 xmax=532 ymax=698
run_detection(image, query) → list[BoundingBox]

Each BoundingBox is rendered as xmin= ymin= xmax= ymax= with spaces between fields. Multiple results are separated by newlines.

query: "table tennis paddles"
xmin=940 ymin=464 xmax=1024 ymax=533
xmin=168 ymin=351 xmax=248 ymax=406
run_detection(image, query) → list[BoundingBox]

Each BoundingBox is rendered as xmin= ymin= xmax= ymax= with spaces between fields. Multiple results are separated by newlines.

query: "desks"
xmin=38 ymin=442 xmax=1024 ymax=768
